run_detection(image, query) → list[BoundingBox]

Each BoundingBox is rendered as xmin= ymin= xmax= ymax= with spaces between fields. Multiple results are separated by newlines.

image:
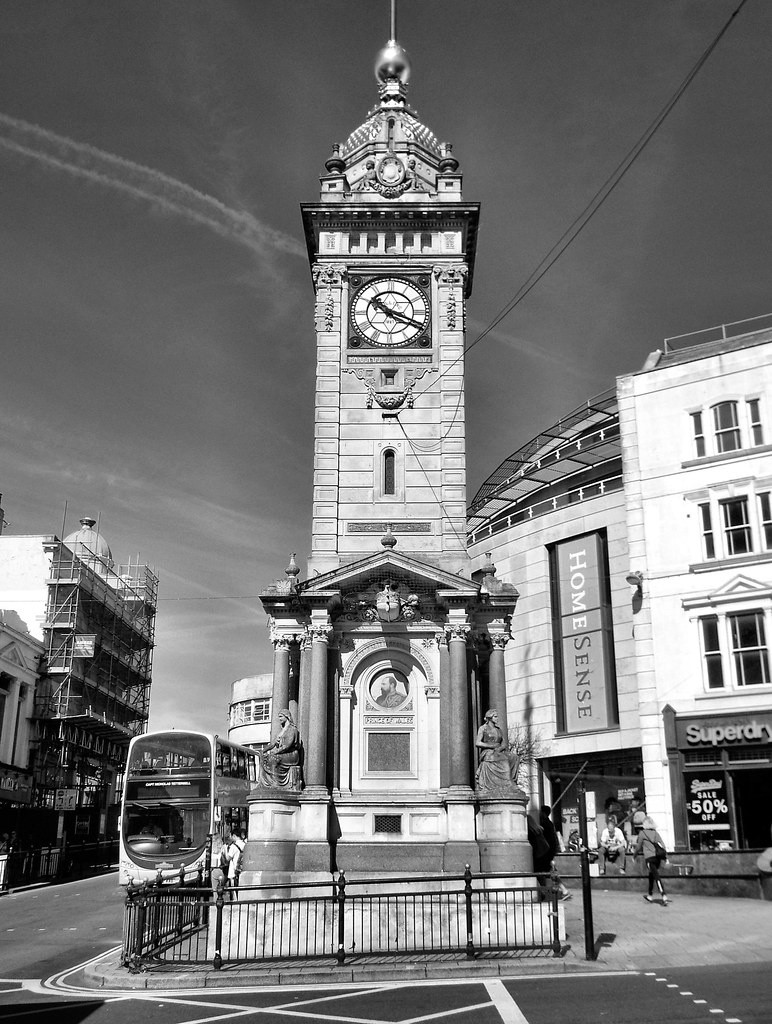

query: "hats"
xmin=541 ymin=805 xmax=551 ymax=816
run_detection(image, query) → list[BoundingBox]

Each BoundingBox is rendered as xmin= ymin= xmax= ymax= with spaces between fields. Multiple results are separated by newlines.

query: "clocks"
xmin=348 ymin=275 xmax=432 ymax=346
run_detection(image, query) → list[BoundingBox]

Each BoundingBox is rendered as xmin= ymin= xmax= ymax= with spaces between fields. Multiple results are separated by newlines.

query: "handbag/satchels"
xmin=642 ymin=830 xmax=666 ymax=859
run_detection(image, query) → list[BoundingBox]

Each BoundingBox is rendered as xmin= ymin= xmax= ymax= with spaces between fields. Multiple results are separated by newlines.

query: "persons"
xmin=475 ymin=709 xmax=519 ymax=791
xmin=631 ymin=816 xmax=669 ymax=906
xmin=211 ymin=830 xmax=247 ymax=903
xmin=255 ymin=709 xmax=299 ymax=789
xmin=527 ymin=806 xmax=571 ymax=902
xmin=0 ymin=828 xmax=23 ymax=884
xmin=598 ymin=821 xmax=627 ymax=875
xmin=376 ymin=676 xmax=407 ymax=708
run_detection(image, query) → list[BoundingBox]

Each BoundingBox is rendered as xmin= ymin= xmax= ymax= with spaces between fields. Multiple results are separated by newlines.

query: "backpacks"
xmin=233 ymin=842 xmax=243 ymax=875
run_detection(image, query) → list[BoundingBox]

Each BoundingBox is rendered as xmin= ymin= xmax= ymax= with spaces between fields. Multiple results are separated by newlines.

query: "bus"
xmin=117 ymin=729 xmax=261 ymax=887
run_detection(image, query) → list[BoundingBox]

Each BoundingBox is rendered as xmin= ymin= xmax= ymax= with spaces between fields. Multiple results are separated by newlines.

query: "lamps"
xmin=626 ymin=570 xmax=645 ymax=598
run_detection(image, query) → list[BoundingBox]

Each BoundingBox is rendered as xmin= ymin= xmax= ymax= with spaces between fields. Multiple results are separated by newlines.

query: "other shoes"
xmin=600 ymin=870 xmax=604 ymax=874
xmin=620 ymin=869 xmax=625 ymax=874
xmin=643 ymin=895 xmax=652 ymax=902
xmin=662 ymin=900 xmax=668 ymax=905
xmin=559 ymin=892 xmax=570 ymax=900
